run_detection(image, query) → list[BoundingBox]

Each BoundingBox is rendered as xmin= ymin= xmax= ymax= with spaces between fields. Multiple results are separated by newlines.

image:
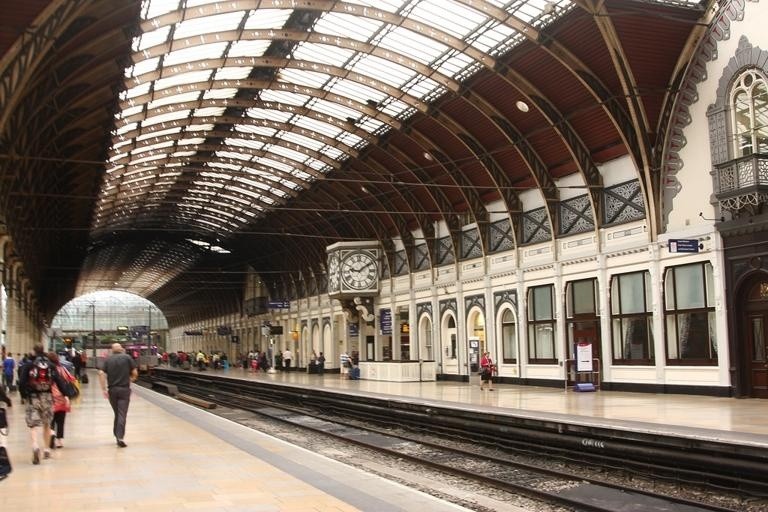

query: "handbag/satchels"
xmin=478 ymin=367 xmax=487 ymax=376
xmin=67 ymin=379 xmax=81 ymax=403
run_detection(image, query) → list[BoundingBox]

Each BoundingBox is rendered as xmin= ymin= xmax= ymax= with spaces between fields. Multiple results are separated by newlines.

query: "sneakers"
xmin=32 ymin=431 xmax=64 ymax=464
xmin=116 ymin=438 xmax=127 ymax=447
xmin=480 ymin=387 xmax=493 ymax=391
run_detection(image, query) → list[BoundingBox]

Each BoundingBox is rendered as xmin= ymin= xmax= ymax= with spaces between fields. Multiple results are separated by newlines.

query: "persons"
xmin=98 ymin=343 xmax=138 ymax=447
xmin=479 ymin=351 xmax=495 ymax=392
xmin=0 ymin=342 xmax=87 ymax=465
xmin=157 ymin=348 xmax=356 ymax=380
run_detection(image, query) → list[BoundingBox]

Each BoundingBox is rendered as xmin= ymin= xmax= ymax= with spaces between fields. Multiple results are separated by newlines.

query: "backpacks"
xmin=30 ymin=356 xmax=54 ymax=392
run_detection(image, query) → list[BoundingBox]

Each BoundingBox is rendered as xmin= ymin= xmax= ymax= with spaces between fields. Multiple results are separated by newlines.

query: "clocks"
xmin=325 ymin=248 xmax=378 ymax=300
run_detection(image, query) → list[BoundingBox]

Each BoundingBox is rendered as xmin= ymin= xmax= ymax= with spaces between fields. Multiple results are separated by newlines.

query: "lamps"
xmin=289 ymin=329 xmax=301 ymax=341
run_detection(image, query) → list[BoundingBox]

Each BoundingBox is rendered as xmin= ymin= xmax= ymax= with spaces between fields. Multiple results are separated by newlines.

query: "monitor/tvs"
xmin=270 ymin=326 xmax=283 ymax=334
xmin=470 ymin=341 xmax=478 ymax=347
xmin=402 ymin=324 xmax=409 ymax=333
